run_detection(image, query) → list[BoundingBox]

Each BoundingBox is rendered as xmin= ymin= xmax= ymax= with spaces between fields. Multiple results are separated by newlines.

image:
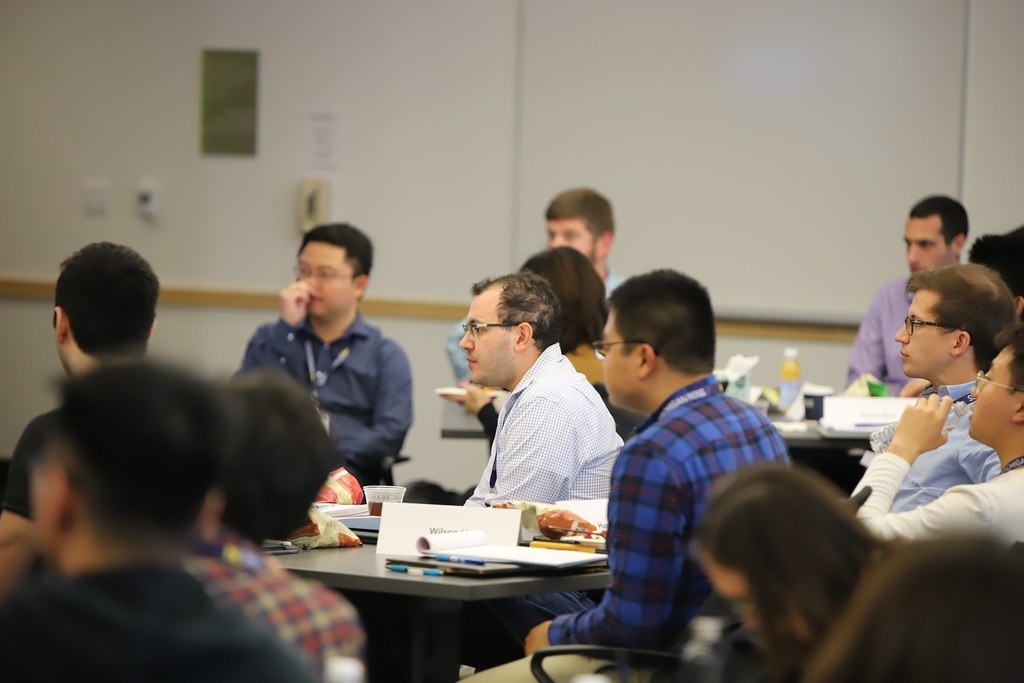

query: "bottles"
xmin=778 ymin=347 xmax=800 ymax=413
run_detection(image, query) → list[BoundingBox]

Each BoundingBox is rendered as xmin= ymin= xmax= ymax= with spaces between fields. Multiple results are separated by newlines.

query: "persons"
xmin=0 ymin=188 xmax=1024 ymax=683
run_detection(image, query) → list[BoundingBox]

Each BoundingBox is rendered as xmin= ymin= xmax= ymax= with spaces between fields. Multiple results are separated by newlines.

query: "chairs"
xmin=530 ymin=479 xmax=873 ymax=683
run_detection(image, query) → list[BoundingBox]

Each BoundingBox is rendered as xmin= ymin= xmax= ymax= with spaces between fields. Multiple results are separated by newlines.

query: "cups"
xmin=363 ymin=485 xmax=407 ymax=516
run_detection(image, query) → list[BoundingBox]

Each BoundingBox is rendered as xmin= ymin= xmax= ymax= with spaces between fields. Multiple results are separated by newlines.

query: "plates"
xmin=560 ymin=534 xmax=606 ymax=551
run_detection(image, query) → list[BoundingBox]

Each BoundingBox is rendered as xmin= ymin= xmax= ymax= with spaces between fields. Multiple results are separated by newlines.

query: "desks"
xmin=272 ymin=544 xmax=611 ymax=682
xmin=771 ymin=413 xmax=888 ymax=454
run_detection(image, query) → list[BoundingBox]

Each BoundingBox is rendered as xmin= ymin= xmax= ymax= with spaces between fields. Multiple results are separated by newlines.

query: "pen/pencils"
xmin=433 ymin=555 xmax=485 ymax=565
xmin=263 ymin=539 xmax=291 ymax=546
xmin=533 ymin=536 xmax=554 ymax=541
xmin=385 ymin=565 xmax=444 ymax=576
xmin=855 ymin=423 xmax=890 ymax=427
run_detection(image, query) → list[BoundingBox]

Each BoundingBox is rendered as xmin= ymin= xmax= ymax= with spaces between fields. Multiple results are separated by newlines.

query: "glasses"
xmin=591 ymin=339 xmax=660 ymax=360
xmin=461 ymin=322 xmax=538 ymax=341
xmin=294 ymin=265 xmax=359 ymax=285
xmin=903 ymin=315 xmax=973 ymax=346
xmin=973 ymin=371 xmax=1024 ymax=398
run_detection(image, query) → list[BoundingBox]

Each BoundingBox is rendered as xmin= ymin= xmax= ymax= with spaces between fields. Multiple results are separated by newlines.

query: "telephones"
xmin=295 ymin=176 xmax=328 ymax=235
